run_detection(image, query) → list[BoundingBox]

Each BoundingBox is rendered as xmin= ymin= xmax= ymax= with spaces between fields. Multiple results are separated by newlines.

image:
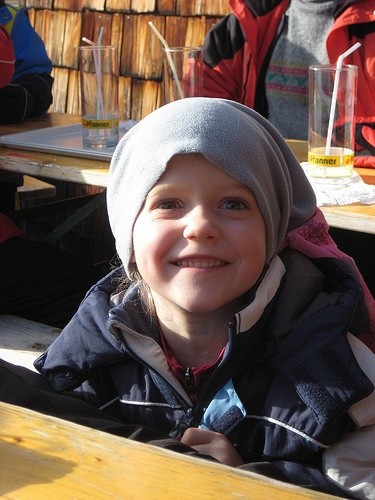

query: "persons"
xmin=0 ymin=97 xmax=375 ymax=500
xmin=0 ymin=0 xmax=55 ymax=231
xmin=180 ymin=0 xmax=375 ymax=300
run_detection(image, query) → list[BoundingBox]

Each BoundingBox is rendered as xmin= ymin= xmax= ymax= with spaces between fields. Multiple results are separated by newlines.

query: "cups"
xmin=163 ymin=47 xmax=202 ymax=105
xmin=79 ymin=47 xmax=120 ymax=145
xmin=309 ymin=64 xmax=356 ymax=176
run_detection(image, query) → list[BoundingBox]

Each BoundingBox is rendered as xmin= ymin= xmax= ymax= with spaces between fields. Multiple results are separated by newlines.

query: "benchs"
xmin=0 ymin=314 xmax=70 ymax=390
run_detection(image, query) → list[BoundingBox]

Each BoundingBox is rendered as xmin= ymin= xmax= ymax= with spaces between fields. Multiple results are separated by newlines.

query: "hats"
xmin=106 ymin=96 xmax=375 ymax=326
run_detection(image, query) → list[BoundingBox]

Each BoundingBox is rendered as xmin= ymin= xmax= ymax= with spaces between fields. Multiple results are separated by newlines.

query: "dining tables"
xmin=0 ymin=111 xmax=375 ymax=292
xmin=0 ymin=401 xmax=349 ymax=500
